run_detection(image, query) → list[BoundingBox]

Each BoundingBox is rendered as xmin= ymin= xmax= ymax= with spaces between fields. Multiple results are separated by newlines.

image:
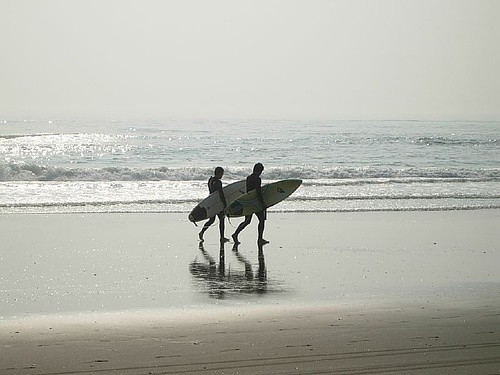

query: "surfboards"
xmin=188 ymin=180 xmax=247 ymax=222
xmin=223 ymin=180 xmax=302 ymax=217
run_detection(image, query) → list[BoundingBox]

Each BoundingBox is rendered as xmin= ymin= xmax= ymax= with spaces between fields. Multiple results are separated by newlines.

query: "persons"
xmin=199 ymin=167 xmax=230 ymax=243
xmin=231 ymin=162 xmax=269 ymax=244
